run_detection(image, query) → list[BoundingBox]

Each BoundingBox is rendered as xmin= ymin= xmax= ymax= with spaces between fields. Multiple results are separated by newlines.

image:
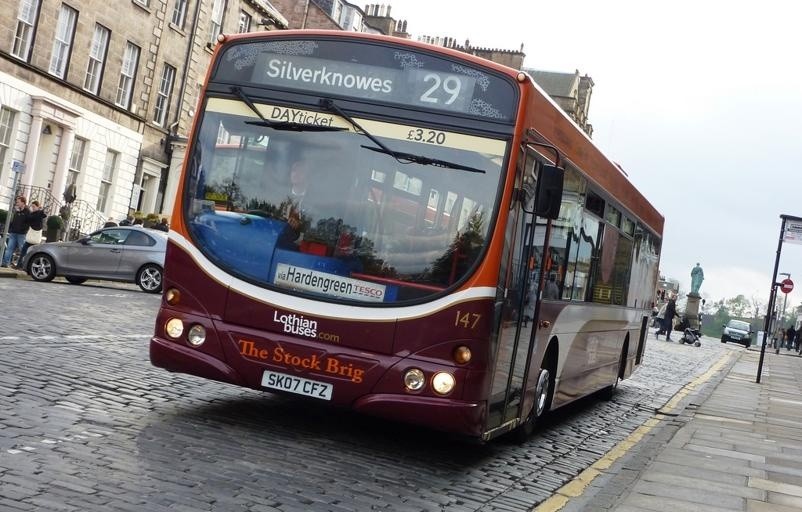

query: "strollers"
xmin=675 ymin=312 xmax=703 ymax=347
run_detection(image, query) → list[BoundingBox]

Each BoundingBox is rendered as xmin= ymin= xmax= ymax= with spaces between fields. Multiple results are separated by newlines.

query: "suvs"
xmin=720 ymin=318 xmax=755 ymax=347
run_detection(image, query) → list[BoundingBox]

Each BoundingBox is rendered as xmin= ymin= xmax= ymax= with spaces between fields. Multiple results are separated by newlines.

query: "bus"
xmin=145 ymin=27 xmax=667 ymax=449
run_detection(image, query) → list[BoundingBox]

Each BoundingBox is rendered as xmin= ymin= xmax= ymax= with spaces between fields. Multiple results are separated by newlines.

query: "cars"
xmin=20 ymin=225 xmax=168 ymax=294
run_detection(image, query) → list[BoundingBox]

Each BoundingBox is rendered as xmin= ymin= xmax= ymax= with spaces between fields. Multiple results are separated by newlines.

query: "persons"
xmin=690 ymin=262 xmax=704 ymax=294
xmin=510 ymin=269 xmax=585 ymax=329
xmin=12 ymin=201 xmax=47 ymax=270
xmin=273 ymin=159 xmax=318 ymax=226
xmin=4 ymin=195 xmax=21 ymax=260
xmin=651 ymin=289 xmax=681 ymax=342
xmin=2 ymin=196 xmax=30 ymax=268
xmin=772 ymin=325 xmax=802 ymax=355
xmin=101 ymin=213 xmax=169 ymax=244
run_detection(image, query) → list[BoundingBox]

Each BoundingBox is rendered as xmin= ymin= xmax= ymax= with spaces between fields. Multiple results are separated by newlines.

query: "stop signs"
xmin=780 ymin=278 xmax=794 ymax=294
